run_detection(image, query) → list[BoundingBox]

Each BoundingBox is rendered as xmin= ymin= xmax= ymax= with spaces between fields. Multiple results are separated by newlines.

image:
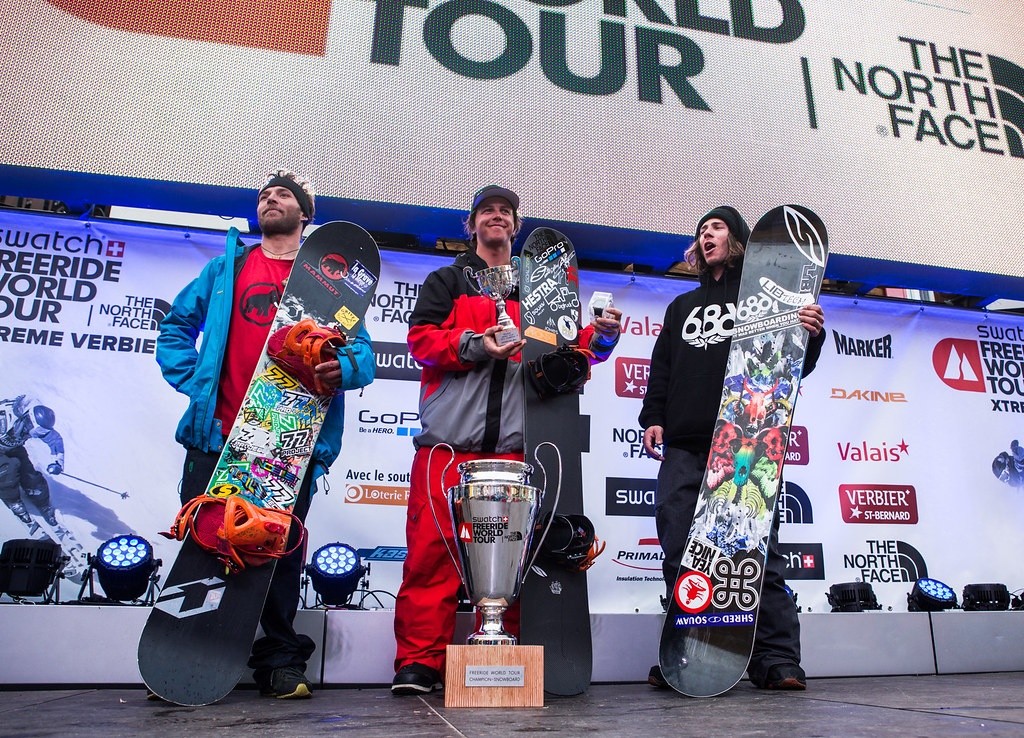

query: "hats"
xmin=697 ymin=208 xmax=742 ymax=241
xmin=472 ymin=184 xmax=518 ymax=210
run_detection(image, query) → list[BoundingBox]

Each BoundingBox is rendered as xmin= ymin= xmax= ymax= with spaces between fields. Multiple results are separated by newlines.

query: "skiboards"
xmin=19 ymin=514 xmax=96 ymax=578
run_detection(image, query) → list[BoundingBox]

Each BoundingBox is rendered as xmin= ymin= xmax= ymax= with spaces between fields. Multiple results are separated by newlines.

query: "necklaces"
xmin=260 ymin=245 xmax=299 ymax=258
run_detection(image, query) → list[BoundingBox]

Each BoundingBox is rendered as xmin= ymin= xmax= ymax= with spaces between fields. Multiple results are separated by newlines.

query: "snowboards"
xmin=655 ymin=199 xmax=833 ymax=700
xmin=135 ymin=215 xmax=384 ymax=709
xmin=514 ymin=225 xmax=595 ymax=701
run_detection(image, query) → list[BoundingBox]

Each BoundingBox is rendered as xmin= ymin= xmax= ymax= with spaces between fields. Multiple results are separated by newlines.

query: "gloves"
xmin=47 ymin=463 xmax=62 ymax=475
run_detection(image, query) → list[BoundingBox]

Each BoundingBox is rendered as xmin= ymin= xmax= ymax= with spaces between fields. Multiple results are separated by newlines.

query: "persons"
xmin=0 ymin=394 xmax=97 ymax=578
xmin=638 ymin=205 xmax=827 ymax=690
xmin=146 ymin=167 xmax=376 ymax=700
xmin=391 ymin=184 xmax=623 ymax=691
xmin=1011 ymin=440 xmax=1024 ymax=486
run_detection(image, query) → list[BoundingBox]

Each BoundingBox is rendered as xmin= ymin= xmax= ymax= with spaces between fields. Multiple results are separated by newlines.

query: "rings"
xmin=813 ymin=319 xmax=819 ymax=326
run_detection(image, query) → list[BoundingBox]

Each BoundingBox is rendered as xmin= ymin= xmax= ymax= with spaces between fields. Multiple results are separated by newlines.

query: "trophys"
xmin=463 ymin=256 xmax=521 ymax=347
xmin=426 ymin=441 xmax=562 ymax=644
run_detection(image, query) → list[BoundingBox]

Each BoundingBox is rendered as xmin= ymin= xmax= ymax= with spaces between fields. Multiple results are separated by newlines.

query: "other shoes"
xmin=769 ymin=663 xmax=807 ymax=689
xmin=40 ymin=503 xmax=58 ymax=525
xmin=5 ymin=497 xmax=26 ymax=515
xmin=649 ymin=664 xmax=669 ymax=688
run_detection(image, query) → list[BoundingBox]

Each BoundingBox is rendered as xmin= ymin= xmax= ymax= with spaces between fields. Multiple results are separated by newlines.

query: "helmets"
xmin=33 ymin=405 xmax=55 ymax=430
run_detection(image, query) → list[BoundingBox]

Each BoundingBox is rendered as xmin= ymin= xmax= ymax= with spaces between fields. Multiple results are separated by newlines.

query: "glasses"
xmin=35 ymin=425 xmax=49 ymax=437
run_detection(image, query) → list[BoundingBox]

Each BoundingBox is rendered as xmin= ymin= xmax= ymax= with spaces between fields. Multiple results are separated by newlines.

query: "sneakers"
xmin=390 ymin=663 xmax=442 ymax=694
xmin=261 ymin=666 xmax=312 ymax=698
